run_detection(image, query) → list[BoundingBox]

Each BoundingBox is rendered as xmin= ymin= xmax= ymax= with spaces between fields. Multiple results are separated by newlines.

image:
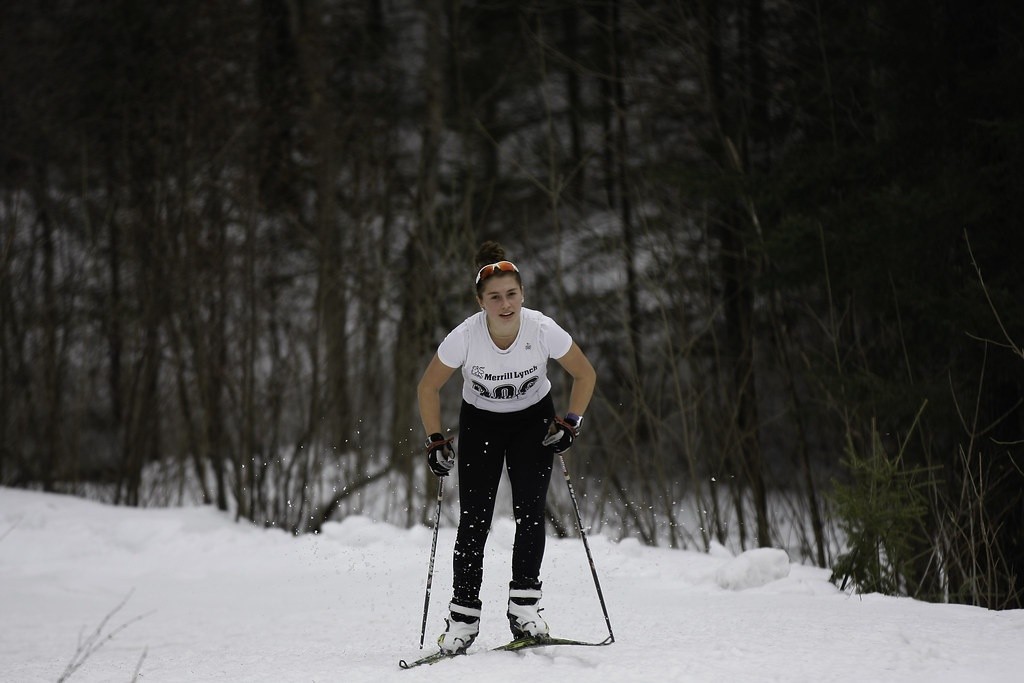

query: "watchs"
xmin=565 ymin=413 xmax=584 ymax=428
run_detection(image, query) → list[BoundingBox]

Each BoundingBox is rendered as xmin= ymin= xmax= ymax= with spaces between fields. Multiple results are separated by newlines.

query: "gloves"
xmin=541 ymin=412 xmax=585 ymax=456
xmin=424 ymin=433 xmax=455 ymax=478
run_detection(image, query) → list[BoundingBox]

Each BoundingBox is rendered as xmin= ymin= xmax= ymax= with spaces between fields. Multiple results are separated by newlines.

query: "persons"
xmin=418 ymin=242 xmax=596 ymax=657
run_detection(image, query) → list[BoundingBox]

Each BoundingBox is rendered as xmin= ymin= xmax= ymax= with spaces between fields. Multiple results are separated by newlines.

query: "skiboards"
xmin=398 ymin=633 xmax=613 ymax=671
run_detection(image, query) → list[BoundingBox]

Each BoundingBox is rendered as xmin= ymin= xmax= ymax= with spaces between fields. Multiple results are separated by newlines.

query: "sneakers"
xmin=437 ymin=596 xmax=482 ymax=655
xmin=507 ymin=579 xmax=550 ymax=640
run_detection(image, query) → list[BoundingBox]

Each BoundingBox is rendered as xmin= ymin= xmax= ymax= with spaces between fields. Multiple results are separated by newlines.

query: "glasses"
xmin=475 ymin=261 xmax=519 ymax=284
